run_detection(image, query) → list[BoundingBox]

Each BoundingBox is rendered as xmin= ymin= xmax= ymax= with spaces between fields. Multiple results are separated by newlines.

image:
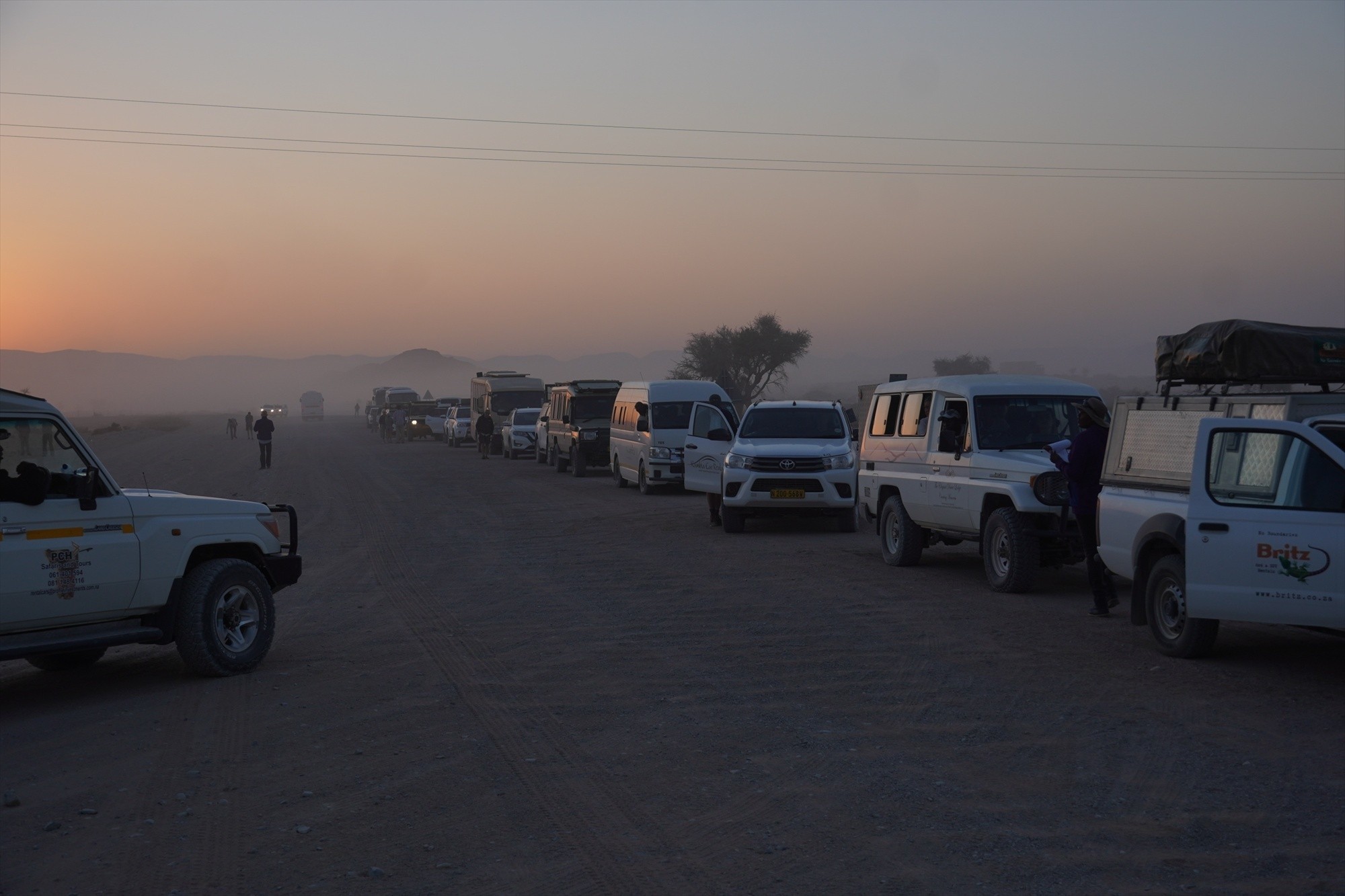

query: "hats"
xmin=1071 ymin=397 xmax=1109 ymax=430
xmin=938 ymin=409 xmax=961 ymax=421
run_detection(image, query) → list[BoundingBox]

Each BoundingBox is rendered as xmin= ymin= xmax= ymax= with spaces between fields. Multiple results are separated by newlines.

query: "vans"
xmin=609 ymin=380 xmax=741 ymax=496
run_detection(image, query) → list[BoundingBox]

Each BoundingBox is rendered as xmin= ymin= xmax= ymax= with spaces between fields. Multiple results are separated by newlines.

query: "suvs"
xmin=260 ymin=404 xmax=275 ymax=417
xmin=366 ymin=386 xmax=470 ymax=441
xmin=543 ymin=379 xmax=622 ymax=476
xmin=535 ymin=401 xmax=551 ymax=463
xmin=1097 ymin=382 xmax=1345 ymax=659
xmin=501 ymin=407 xmax=542 ymax=460
xmin=857 ymin=373 xmax=1113 ymax=590
xmin=684 ymin=399 xmax=863 ymax=533
xmin=0 ymin=385 xmax=303 ymax=677
xmin=274 ymin=404 xmax=288 ymax=417
xmin=447 ymin=403 xmax=472 ymax=448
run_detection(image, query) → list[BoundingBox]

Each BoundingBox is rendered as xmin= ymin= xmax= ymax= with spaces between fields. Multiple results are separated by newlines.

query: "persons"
xmin=1049 ymin=396 xmax=1124 ymax=619
xmin=245 ymin=411 xmax=254 ymax=440
xmin=634 ymin=401 xmax=686 ymax=431
xmin=918 ymin=396 xmax=1013 ymax=453
xmin=694 ymin=394 xmax=738 ymax=528
xmin=226 ymin=418 xmax=238 ymax=440
xmin=0 ymin=428 xmax=38 ymax=502
xmin=15 ymin=418 xmax=33 ymax=456
xmin=253 ymin=410 xmax=275 ymax=470
xmin=39 ymin=418 xmax=57 ymax=458
xmin=366 ymin=404 xmax=407 ymax=442
xmin=476 ymin=410 xmax=494 ymax=459
xmin=822 ymin=412 xmax=844 ymax=437
xmin=354 ymin=403 xmax=360 ymax=416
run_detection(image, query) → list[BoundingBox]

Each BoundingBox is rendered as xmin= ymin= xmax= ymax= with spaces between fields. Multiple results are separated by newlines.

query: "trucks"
xmin=299 ymin=391 xmax=325 ymax=421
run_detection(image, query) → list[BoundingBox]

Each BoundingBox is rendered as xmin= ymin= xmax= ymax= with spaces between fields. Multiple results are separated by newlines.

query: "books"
xmin=1043 ymin=439 xmax=1071 ymax=463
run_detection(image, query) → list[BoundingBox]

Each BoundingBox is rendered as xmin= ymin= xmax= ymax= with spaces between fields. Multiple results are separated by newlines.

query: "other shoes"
xmin=1089 ymin=580 xmax=1121 ymax=616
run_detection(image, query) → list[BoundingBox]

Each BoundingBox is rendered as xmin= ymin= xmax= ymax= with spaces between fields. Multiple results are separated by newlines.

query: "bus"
xmin=469 ymin=370 xmax=544 ymax=456
